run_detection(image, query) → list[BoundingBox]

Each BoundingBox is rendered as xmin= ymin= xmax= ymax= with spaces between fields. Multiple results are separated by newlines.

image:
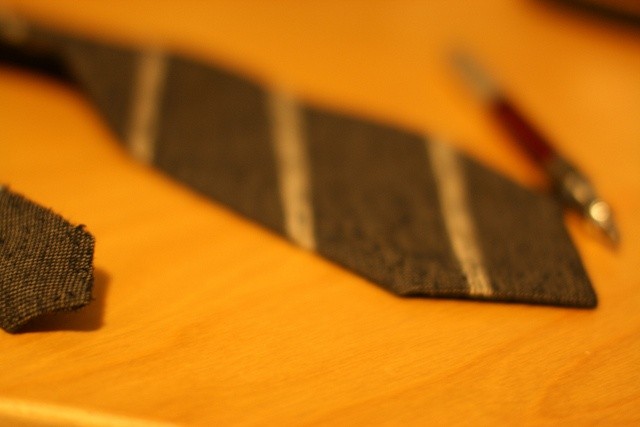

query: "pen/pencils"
xmin=484 ymin=89 xmax=620 ymax=248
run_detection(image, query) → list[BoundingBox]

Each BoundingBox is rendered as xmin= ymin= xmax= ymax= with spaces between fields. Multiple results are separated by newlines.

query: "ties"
xmin=1 ymin=26 xmax=596 ymax=334
